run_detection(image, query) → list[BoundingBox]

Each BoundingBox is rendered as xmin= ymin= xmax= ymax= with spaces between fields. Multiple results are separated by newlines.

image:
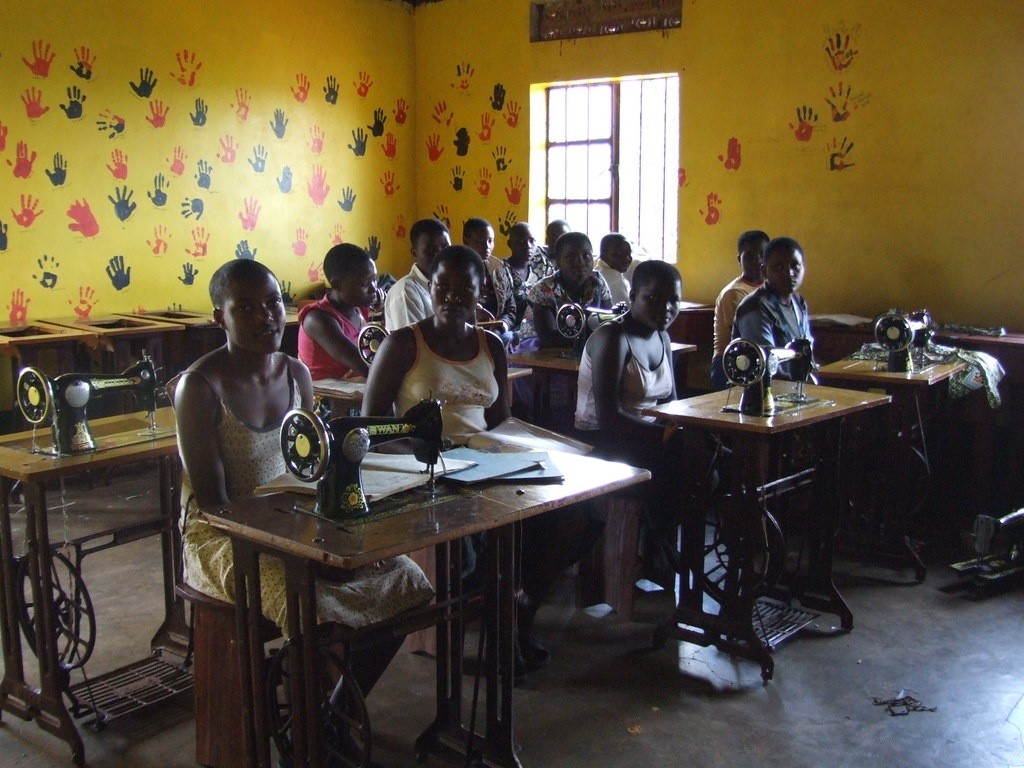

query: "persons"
xmin=297 ymin=243 xmax=377 ymax=380
xmin=361 ymin=245 xmax=607 ymax=684
xmin=730 ymin=236 xmax=926 ymax=550
xmin=593 ymin=234 xmax=632 ymax=304
xmin=573 ymin=261 xmax=731 ymax=495
xmin=384 ymin=219 xmax=451 ymax=334
xmin=711 ymin=230 xmax=769 ymax=388
xmin=506 ymin=219 xmax=557 ymax=331
xmin=543 ymin=220 xmax=570 ymax=256
xmin=163 ymin=259 xmax=438 ymax=768
xmin=462 ymin=218 xmax=517 ymax=343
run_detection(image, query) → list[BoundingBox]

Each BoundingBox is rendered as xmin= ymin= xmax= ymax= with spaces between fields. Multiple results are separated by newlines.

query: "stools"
xmin=175 ymin=582 xmax=284 ymax=767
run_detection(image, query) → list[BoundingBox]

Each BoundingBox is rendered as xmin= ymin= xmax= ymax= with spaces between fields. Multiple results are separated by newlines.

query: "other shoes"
xmin=515 ymin=640 xmax=529 ymax=680
xmin=320 ymin=700 xmax=384 ymax=768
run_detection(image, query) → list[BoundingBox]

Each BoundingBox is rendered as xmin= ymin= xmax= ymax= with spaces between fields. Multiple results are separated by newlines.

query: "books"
xmin=254 ymin=447 xmax=566 ymax=502
xmin=311 ymin=379 xmax=367 ymax=400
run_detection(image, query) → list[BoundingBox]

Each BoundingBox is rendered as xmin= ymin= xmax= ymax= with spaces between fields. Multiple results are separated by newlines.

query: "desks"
xmin=669 ymin=308 xmax=1024 ymax=513
